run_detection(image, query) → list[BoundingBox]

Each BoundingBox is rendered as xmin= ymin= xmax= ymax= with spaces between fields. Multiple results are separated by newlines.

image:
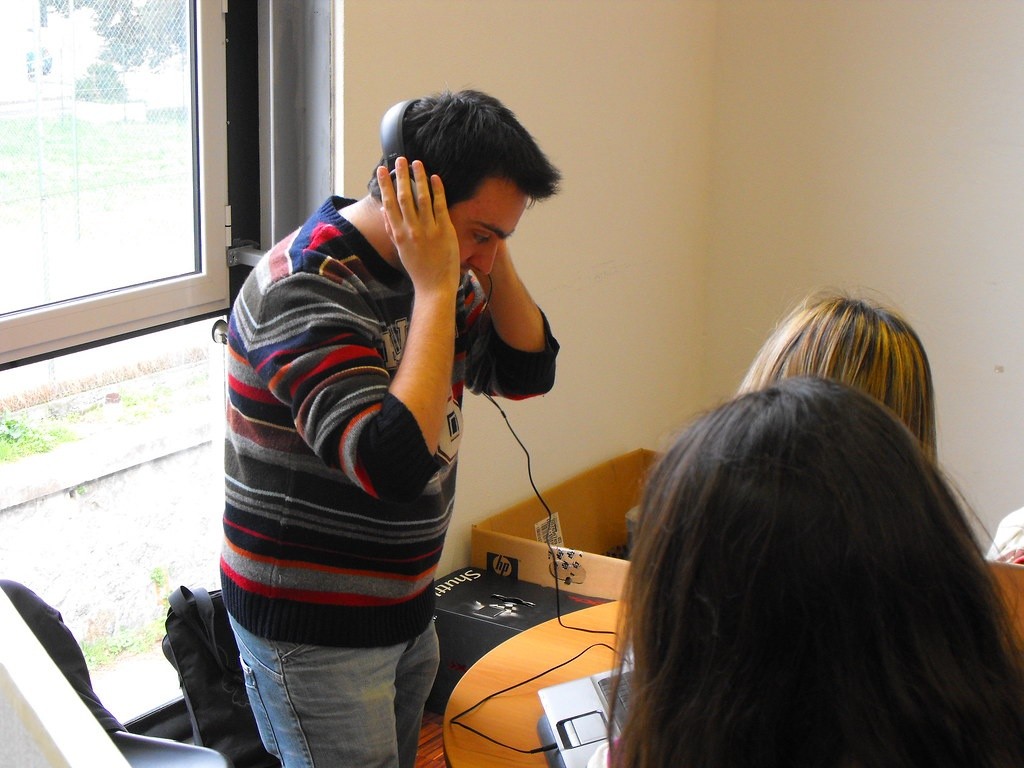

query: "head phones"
xmin=380 ymin=99 xmax=426 ymax=212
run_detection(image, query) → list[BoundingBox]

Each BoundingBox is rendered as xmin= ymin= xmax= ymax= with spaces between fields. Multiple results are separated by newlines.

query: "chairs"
xmin=0 ymin=581 xmax=232 ymax=768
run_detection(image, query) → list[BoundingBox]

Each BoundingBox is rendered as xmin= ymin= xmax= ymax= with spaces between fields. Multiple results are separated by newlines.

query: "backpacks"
xmin=161 ymin=584 xmax=275 ymax=767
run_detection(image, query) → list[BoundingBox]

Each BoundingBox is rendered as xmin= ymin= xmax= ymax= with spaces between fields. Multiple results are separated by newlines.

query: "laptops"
xmin=536 ymin=653 xmax=639 ymax=768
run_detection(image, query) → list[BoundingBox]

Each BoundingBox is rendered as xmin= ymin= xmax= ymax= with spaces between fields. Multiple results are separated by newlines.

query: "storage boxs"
xmin=468 ymin=448 xmax=664 ymax=600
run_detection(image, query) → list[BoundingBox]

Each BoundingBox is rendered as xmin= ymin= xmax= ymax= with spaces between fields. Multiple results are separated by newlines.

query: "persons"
xmin=723 ymin=293 xmax=937 ymax=467
xmin=216 ymin=89 xmax=560 ymax=768
xmin=580 ymin=378 xmax=1023 ymax=768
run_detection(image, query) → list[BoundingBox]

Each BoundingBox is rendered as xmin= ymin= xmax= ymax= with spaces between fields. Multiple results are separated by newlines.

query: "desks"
xmin=439 ymin=556 xmax=1023 ymax=767
